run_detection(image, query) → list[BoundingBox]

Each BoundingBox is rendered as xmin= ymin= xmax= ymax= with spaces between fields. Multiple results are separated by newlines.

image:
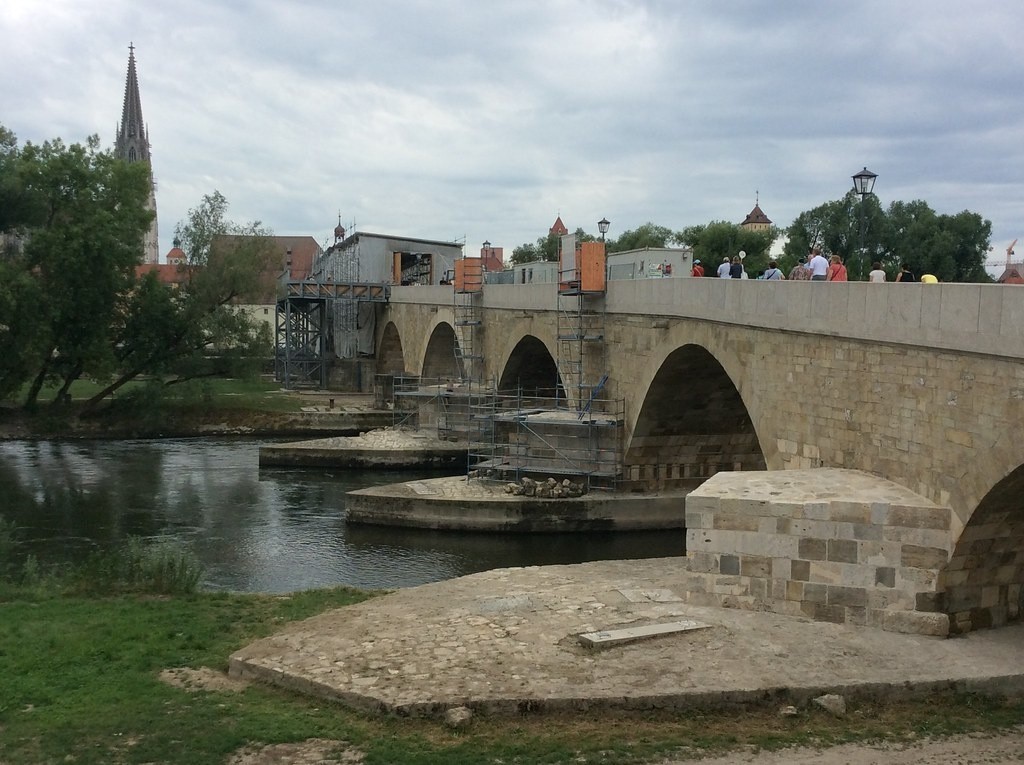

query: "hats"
xmin=692 ymin=260 xmax=701 ymax=264
xmin=723 ymin=257 xmax=730 ymax=262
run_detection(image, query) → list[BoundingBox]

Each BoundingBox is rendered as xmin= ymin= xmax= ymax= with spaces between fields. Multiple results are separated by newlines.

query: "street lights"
xmin=483 ymin=241 xmax=491 ymax=272
xmin=851 ymin=166 xmax=880 ymax=281
xmin=597 ymin=217 xmax=611 ymax=280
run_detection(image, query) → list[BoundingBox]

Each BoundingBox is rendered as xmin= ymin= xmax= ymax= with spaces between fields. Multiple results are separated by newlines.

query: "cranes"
xmin=1006 ymin=238 xmax=1018 ymax=270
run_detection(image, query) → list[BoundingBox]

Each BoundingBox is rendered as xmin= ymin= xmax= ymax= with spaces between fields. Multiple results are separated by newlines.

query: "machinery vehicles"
xmin=440 ymin=268 xmax=454 ymax=284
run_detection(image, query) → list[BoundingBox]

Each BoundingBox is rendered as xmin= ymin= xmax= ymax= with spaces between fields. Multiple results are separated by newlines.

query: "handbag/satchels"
xmin=740 ymin=265 xmax=748 ymax=279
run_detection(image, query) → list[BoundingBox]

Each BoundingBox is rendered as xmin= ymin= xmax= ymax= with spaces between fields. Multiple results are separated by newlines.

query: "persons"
xmin=690 ymin=259 xmax=704 ymax=277
xmin=893 ymin=263 xmax=915 ymax=283
xmin=717 ymin=255 xmax=743 ymax=279
xmin=868 ymin=262 xmax=886 ymax=283
xmin=762 ymin=247 xmax=847 ymax=282
